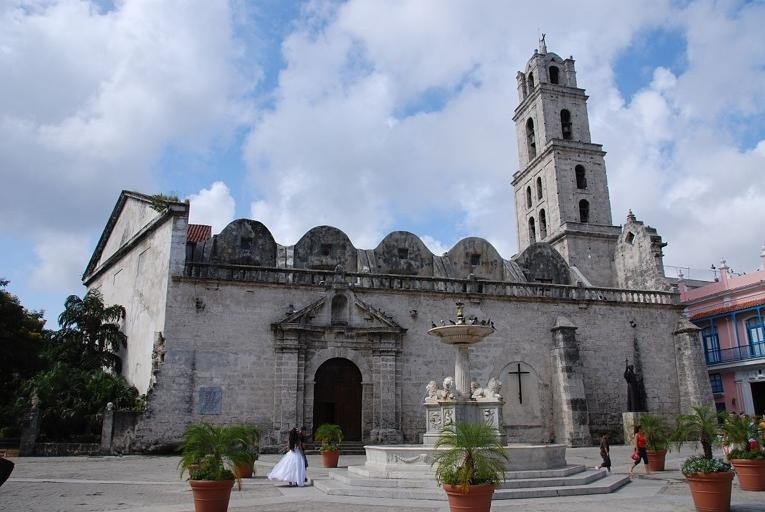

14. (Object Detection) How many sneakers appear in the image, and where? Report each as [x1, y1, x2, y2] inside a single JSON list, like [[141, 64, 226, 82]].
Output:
[[594, 465, 600, 469]]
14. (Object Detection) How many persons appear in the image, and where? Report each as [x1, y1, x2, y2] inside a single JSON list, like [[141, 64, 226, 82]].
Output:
[[267, 427, 306, 486], [623, 364, 644, 411], [721, 410, 765, 470], [595, 431, 612, 471], [629, 425, 650, 474], [298, 425, 308, 482]]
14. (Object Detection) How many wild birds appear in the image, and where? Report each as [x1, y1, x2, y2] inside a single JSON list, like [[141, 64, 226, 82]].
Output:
[[432, 317, 496, 331]]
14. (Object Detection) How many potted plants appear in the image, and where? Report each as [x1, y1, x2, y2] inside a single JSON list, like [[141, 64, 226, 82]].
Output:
[[315, 420, 345, 467], [427, 413, 512, 512], [175, 418, 261, 512], [637, 397, 764, 512]]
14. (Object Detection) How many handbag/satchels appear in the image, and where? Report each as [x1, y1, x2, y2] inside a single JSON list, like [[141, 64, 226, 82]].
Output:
[[631, 452, 641, 461]]
